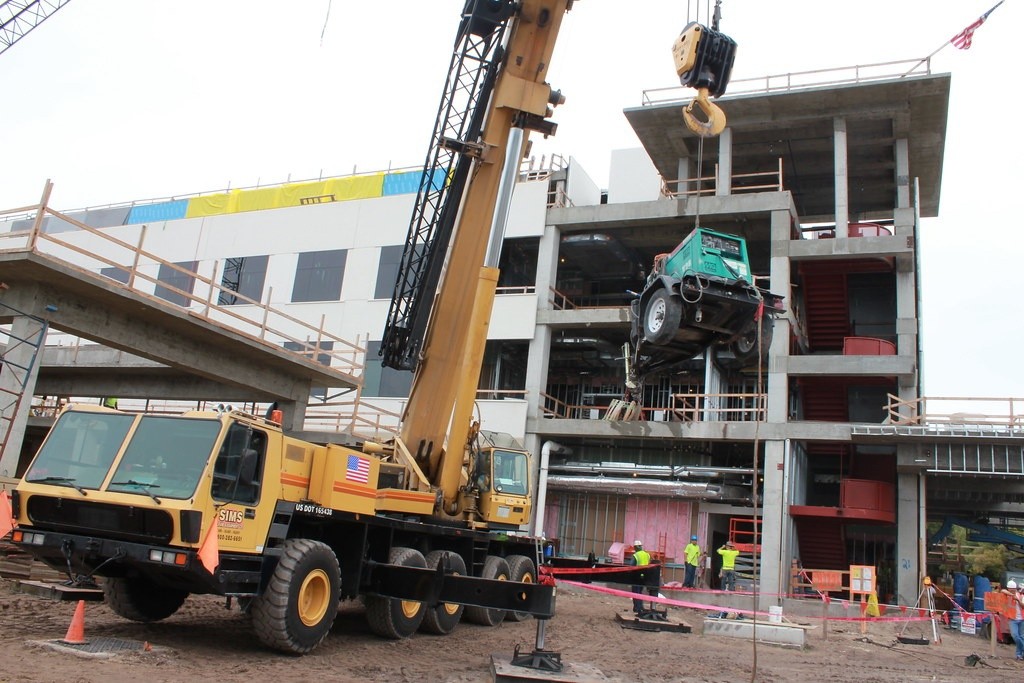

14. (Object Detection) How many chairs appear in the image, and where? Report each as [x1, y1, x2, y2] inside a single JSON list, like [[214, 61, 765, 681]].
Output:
[[216, 455, 256, 504]]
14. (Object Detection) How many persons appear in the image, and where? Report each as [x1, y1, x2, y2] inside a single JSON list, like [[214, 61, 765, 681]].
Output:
[[717, 542, 740, 591], [630, 541, 650, 612], [1001, 580, 1024, 659], [682, 535, 707, 589]]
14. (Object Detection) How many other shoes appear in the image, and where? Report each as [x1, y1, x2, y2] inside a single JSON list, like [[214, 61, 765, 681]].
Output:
[[1017, 655, 1023, 660]]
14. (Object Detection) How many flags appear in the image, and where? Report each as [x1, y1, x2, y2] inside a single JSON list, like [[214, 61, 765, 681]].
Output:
[[950, 1, 1006, 50]]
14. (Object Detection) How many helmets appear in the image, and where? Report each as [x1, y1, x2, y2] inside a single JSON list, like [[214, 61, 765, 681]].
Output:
[[633, 541, 642, 546], [1007, 580, 1017, 589], [691, 535, 697, 540], [725, 542, 734, 547]]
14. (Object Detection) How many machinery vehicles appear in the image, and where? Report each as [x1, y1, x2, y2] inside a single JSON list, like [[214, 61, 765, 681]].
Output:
[[613, 225, 791, 404], [6, 0, 737, 675]]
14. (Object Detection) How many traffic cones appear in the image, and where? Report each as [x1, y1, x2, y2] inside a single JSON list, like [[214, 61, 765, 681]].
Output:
[[57, 599, 91, 646]]
